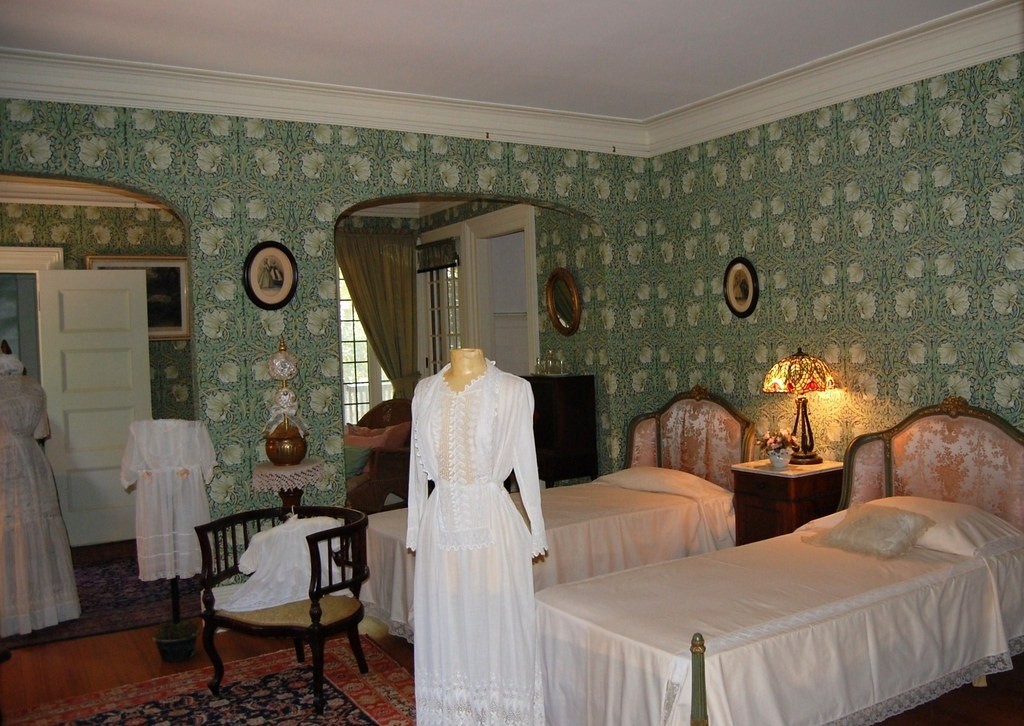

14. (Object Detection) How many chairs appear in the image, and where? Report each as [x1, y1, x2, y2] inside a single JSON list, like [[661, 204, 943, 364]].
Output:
[[348, 397, 412, 512], [195, 503, 372, 716]]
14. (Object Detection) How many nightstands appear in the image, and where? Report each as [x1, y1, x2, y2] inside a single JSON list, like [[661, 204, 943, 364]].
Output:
[[730, 456, 842, 549]]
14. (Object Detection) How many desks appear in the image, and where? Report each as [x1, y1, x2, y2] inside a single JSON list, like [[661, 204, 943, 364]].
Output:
[[254, 457, 325, 523]]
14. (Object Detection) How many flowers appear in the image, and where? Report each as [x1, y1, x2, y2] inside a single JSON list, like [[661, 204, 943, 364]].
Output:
[[754, 425, 797, 458]]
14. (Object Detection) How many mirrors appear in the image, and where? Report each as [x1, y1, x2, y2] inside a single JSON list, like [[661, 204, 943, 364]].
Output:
[[544, 266, 583, 337]]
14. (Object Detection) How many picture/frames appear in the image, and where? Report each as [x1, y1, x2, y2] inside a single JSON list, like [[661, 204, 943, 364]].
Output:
[[242, 240, 299, 311], [87, 254, 192, 342], [722, 255, 760, 320]]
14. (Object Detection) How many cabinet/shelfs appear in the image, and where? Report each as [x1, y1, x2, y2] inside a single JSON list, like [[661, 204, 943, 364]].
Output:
[[519, 373, 601, 487]]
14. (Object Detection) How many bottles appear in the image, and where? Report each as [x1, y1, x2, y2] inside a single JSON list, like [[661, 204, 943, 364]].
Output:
[[536, 350, 569, 374], [263, 414, 310, 466]]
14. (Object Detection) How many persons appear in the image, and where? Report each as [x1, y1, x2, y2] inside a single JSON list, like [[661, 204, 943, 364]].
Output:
[[406, 348, 548, 726], [0, 354, 81, 663], [269, 260, 283, 286], [258, 257, 274, 288]]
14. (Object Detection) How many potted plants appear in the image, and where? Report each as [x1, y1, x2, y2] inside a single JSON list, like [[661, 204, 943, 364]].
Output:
[[149, 619, 199, 664]]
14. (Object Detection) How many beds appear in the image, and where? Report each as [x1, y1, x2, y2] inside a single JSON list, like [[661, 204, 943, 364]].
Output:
[[535, 396, 1024, 726], [364, 382, 758, 649]]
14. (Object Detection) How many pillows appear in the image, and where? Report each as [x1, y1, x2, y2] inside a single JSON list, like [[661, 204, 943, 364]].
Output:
[[593, 465, 727, 502], [345, 433, 388, 473], [346, 422, 411, 448], [802, 500, 936, 560], [344, 443, 374, 479], [795, 494, 1024, 558]]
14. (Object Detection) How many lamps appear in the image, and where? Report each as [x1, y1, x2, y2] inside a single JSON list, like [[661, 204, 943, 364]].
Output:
[[263, 336, 309, 467], [762, 346, 837, 465]]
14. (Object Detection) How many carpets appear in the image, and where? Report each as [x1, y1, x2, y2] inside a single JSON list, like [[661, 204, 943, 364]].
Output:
[[0, 632, 417, 726]]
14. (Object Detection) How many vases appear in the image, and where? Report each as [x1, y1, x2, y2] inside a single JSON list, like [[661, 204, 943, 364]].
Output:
[[769, 447, 793, 468]]
